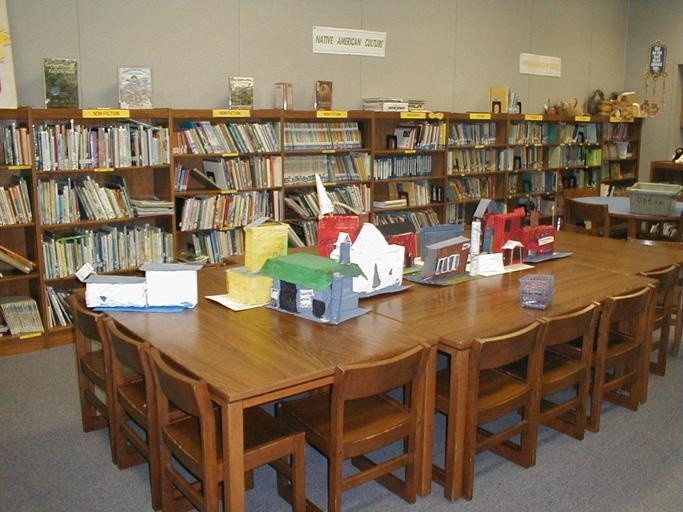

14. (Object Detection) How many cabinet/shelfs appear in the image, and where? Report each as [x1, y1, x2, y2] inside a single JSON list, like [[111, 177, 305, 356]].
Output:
[[446, 113, 507, 229], [282, 110, 373, 248], [170, 107, 283, 269], [560, 117, 602, 190], [0, 110, 175, 356], [600, 118, 641, 191], [371, 111, 447, 230], [649, 159, 683, 184], [505, 115, 561, 226]]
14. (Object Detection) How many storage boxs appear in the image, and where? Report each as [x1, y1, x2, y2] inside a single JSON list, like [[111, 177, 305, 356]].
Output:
[[625, 181, 683, 217]]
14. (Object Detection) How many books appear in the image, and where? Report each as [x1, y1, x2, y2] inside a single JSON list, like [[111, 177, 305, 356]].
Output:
[[548, 146, 602, 168], [374, 208, 440, 230], [0, 295, 44, 338], [76, 209, 573, 325], [392, 120, 446, 150], [561, 122, 602, 143], [610, 163, 623, 180], [172, 121, 281, 155], [509, 121, 558, 145], [561, 167, 598, 189], [446, 203, 466, 226], [284, 184, 371, 218], [117, 65, 152, 110], [284, 152, 371, 186], [176, 228, 244, 265], [447, 175, 495, 202], [507, 147, 543, 171], [607, 124, 633, 140], [43, 58, 79, 108], [33, 119, 170, 171], [448, 122, 496, 145], [276, 82, 292, 109], [228, 76, 255, 110], [373, 181, 444, 209], [0, 122, 31, 166], [447, 149, 506, 173], [37, 175, 174, 225], [174, 190, 279, 233], [362, 98, 428, 113], [42, 221, 173, 282], [0, 245, 36, 278], [0, 175, 32, 225], [614, 141, 634, 158], [373, 154, 432, 180], [489, 87, 521, 114], [508, 171, 558, 195], [283, 122, 362, 152], [315, 81, 333, 111], [175, 154, 283, 192], [517, 195, 556, 216], [46, 285, 72, 329], [286, 220, 318, 248]]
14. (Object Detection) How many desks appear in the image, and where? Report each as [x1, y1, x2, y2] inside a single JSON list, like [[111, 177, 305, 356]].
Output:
[[73, 264, 439, 512], [555, 231, 682, 391], [360, 257, 659, 503], [573, 196, 683, 240]]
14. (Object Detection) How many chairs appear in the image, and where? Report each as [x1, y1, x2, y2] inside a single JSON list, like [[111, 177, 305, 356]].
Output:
[[145, 347, 305, 512], [560, 199, 612, 235], [403, 318, 545, 500], [68, 294, 142, 466], [670, 261, 683, 358], [476, 301, 601, 468], [275, 340, 431, 512], [102, 316, 254, 512], [638, 261, 679, 377], [542, 284, 655, 433]]
